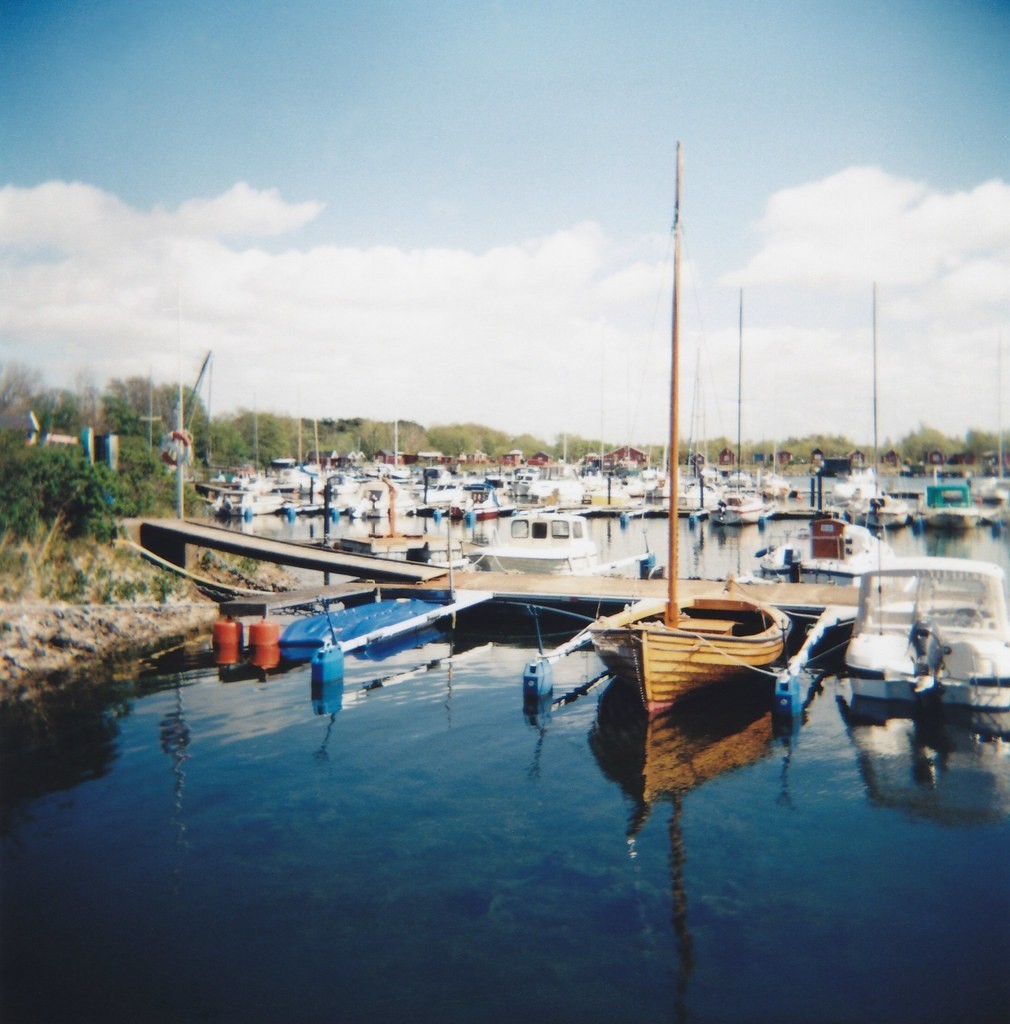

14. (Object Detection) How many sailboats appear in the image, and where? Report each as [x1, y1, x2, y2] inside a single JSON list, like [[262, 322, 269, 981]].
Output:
[[585, 136, 794, 715], [587, 666, 808, 1023]]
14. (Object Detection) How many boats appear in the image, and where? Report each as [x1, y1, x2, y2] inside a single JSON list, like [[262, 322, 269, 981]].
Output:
[[460, 510, 649, 578], [754, 517, 895, 587], [844, 558, 1010, 711], [211, 283, 1009, 533]]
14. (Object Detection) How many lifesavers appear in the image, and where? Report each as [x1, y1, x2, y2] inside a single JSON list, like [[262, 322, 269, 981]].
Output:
[[159, 431, 191, 465]]
